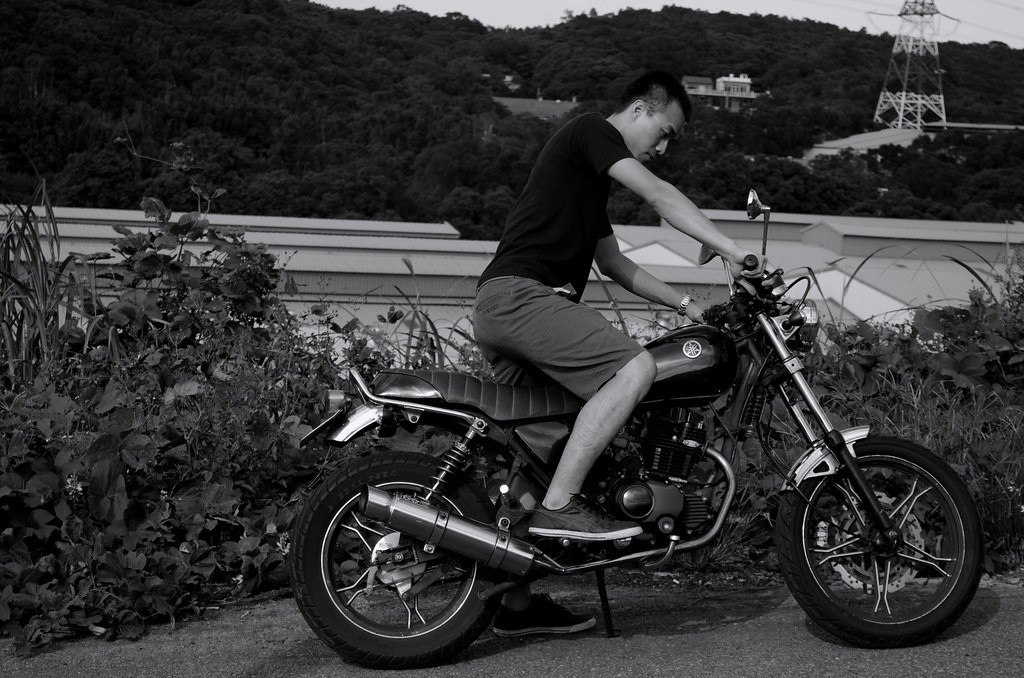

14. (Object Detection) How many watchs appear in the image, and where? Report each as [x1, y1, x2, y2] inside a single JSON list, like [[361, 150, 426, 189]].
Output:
[[677, 294, 695, 317]]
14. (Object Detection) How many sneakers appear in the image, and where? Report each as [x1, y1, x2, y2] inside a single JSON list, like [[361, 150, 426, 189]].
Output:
[[529, 493, 644, 541], [492, 593, 597, 638]]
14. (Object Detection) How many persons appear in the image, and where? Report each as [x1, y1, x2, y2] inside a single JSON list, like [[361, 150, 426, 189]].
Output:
[[473, 70, 768, 638]]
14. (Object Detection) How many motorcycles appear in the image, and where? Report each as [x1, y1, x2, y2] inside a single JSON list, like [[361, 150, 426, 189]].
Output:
[[288, 188, 985, 670]]
